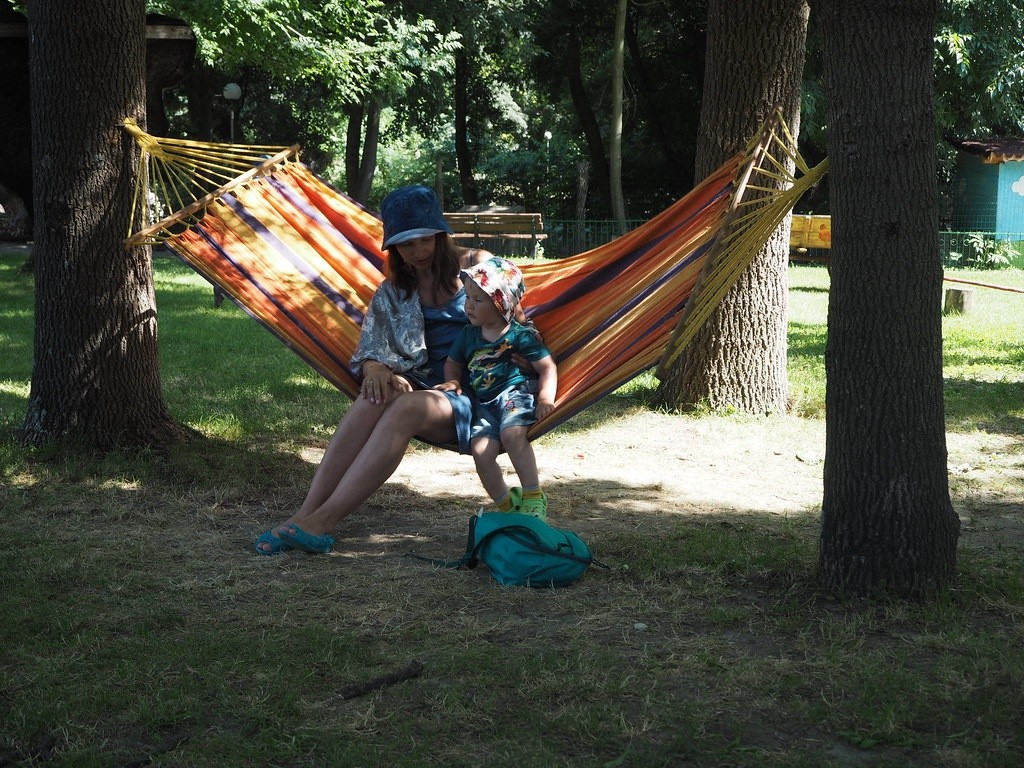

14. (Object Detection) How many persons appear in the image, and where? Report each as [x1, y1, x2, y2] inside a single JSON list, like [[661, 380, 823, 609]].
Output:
[[256, 186, 557, 555]]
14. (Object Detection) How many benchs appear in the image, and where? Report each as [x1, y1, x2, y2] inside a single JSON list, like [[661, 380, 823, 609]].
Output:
[[442, 212, 548, 259], [789, 214, 832, 263]]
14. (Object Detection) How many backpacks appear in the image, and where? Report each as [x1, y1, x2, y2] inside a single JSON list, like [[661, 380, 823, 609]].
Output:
[[456, 512, 593, 587]]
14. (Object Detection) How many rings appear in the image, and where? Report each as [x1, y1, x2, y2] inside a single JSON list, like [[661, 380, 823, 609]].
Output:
[[366, 380, 372, 384]]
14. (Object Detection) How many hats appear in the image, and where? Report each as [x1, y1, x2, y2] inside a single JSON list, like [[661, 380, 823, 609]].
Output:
[[381, 185, 454, 251], [460, 256, 525, 325]]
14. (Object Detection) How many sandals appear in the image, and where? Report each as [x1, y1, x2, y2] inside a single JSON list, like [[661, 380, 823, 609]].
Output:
[[517, 493, 547, 521], [279, 523, 335, 553], [255, 529, 285, 554], [498, 487, 522, 512]]
